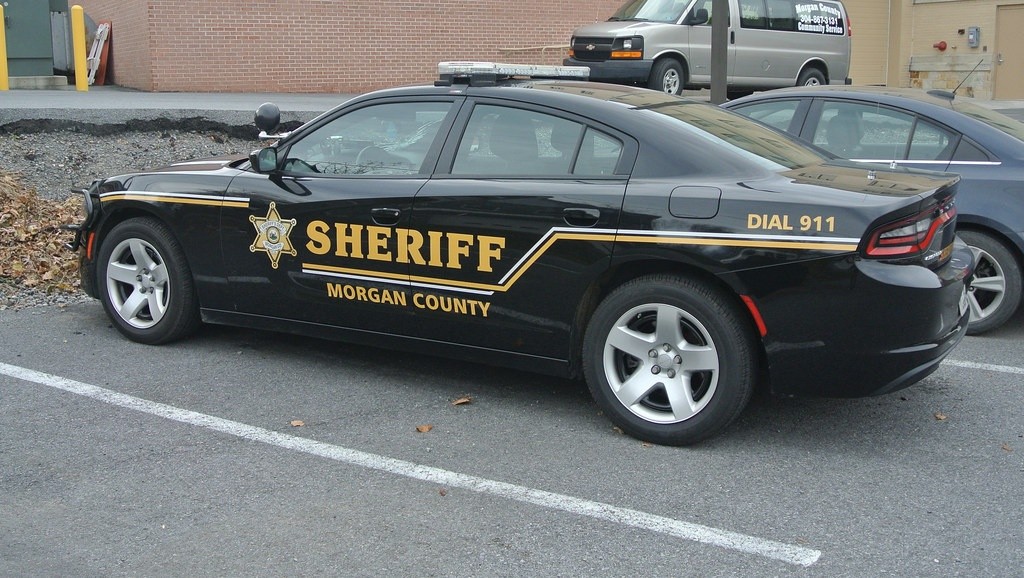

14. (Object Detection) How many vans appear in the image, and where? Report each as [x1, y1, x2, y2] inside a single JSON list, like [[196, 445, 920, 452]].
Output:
[[561, 0, 854, 116]]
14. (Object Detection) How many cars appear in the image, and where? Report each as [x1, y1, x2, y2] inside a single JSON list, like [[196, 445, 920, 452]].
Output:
[[710, 85, 1023, 337], [73, 58, 978, 447]]
